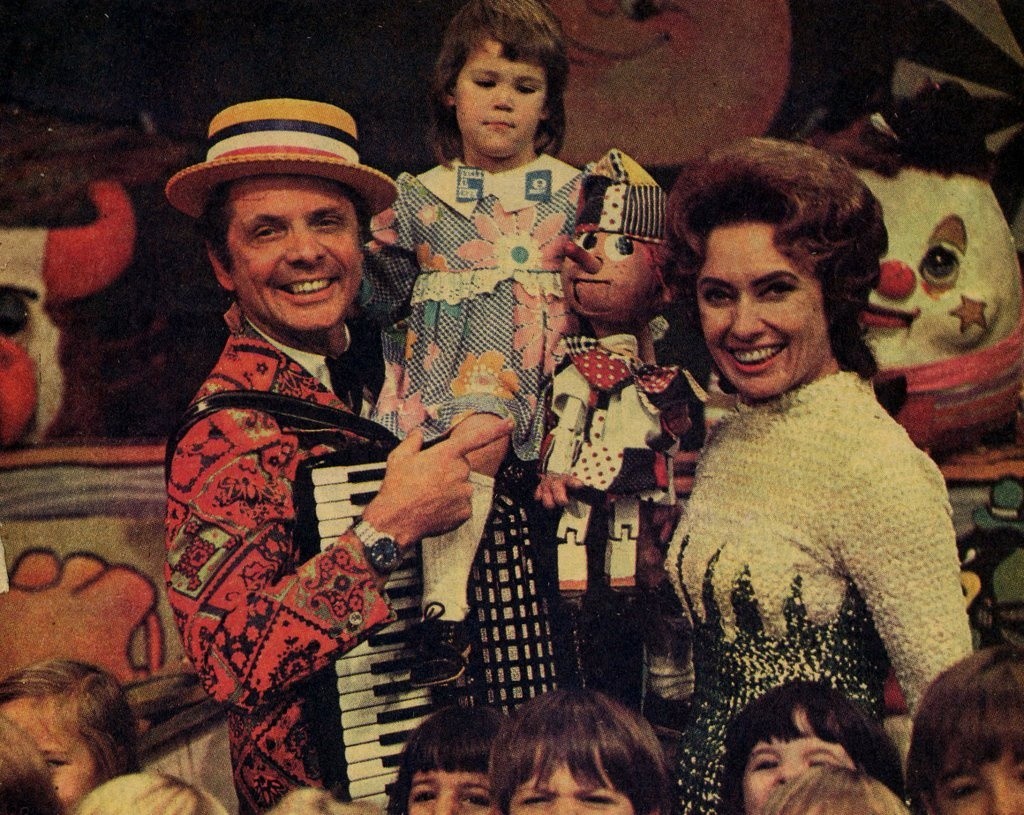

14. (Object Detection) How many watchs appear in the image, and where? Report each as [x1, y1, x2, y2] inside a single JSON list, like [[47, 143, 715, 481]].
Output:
[[351, 517, 406, 573]]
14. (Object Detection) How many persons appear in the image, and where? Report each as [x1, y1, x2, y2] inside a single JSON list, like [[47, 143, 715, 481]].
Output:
[[0, 82, 1024, 815], [225, 0, 691, 687], [531, 148, 686, 600]]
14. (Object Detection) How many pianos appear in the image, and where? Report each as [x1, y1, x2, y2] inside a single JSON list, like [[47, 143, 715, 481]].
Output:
[[292, 439, 584, 814]]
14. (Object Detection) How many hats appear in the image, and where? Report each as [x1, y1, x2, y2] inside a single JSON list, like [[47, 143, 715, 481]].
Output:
[[165, 97, 398, 219]]
